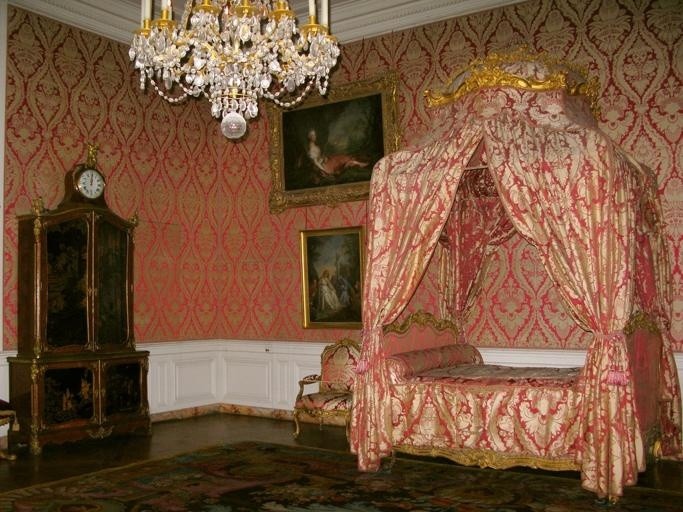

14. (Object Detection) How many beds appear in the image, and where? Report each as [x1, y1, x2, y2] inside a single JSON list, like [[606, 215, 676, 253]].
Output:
[[364, 310, 665, 507]]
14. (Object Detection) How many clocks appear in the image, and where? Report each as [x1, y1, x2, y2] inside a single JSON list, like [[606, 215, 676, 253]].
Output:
[[63, 142, 107, 207]]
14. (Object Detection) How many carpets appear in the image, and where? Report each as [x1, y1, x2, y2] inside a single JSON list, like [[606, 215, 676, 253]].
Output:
[[0, 438, 683, 512]]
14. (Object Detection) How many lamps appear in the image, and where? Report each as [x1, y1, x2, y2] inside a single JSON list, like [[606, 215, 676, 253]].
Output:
[[129, 0, 340, 141]]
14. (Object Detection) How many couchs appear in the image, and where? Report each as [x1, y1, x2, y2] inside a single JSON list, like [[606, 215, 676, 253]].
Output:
[[292, 336, 361, 442]]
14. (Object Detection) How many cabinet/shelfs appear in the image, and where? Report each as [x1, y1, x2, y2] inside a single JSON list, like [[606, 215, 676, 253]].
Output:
[[9, 194, 154, 456]]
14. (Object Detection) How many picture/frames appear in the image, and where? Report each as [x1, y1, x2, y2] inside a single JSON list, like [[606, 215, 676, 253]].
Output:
[[265, 71, 402, 215], [299, 226, 364, 330]]
